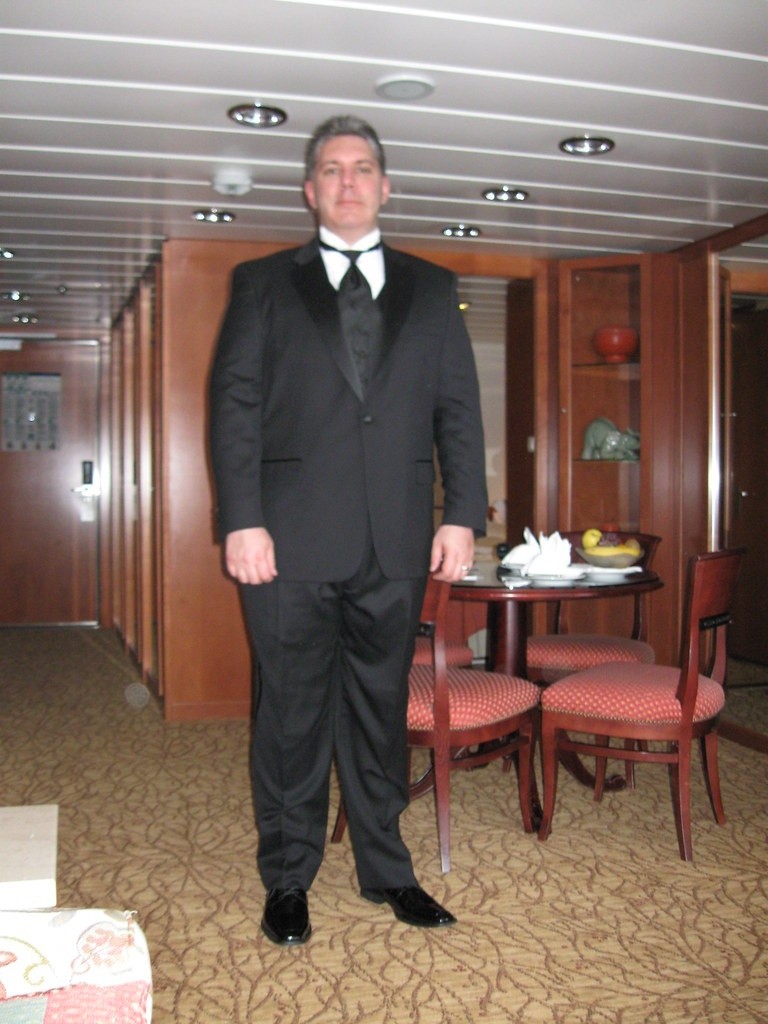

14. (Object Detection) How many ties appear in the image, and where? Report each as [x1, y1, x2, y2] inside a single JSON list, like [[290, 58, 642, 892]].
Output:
[[320, 239, 383, 395]]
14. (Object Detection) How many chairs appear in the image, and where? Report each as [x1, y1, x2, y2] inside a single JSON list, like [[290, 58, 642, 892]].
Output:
[[331, 530, 750, 865], [0, 907, 155, 1024]]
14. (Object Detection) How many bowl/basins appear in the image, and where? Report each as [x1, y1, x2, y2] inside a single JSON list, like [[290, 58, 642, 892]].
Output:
[[575, 547, 644, 566]]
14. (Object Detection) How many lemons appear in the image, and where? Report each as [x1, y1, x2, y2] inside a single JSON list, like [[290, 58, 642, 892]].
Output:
[[585, 529, 601, 546]]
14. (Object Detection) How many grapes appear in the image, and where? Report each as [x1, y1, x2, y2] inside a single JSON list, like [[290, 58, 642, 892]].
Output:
[[598, 531, 619, 548]]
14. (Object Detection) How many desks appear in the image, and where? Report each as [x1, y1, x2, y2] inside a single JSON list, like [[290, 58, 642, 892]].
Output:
[[441, 557, 664, 837]]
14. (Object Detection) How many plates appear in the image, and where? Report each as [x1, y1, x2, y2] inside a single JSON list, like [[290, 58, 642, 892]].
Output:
[[572, 562, 643, 581], [528, 573, 586, 588]]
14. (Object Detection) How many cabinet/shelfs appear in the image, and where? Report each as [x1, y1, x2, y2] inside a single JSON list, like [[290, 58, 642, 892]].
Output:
[[556, 253, 653, 653]]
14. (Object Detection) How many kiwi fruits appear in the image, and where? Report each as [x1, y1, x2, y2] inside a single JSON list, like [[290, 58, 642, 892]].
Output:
[[626, 539, 639, 547]]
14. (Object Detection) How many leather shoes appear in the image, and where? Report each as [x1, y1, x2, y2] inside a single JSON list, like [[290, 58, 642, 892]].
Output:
[[360, 884, 458, 928], [261, 887, 311, 945]]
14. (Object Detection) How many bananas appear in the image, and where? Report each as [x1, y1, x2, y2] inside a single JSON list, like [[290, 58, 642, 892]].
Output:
[[585, 545, 638, 557]]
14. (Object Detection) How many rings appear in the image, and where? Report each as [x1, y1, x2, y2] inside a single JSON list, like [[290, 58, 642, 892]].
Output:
[[461, 565, 469, 571]]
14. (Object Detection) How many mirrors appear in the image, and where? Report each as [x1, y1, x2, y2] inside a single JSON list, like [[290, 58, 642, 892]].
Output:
[[707, 232, 768, 742]]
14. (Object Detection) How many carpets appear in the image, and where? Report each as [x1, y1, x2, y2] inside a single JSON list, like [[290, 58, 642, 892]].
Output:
[[0, 803, 59, 909]]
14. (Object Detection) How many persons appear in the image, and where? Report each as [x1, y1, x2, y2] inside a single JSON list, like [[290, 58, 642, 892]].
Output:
[[206, 114, 490, 945]]
